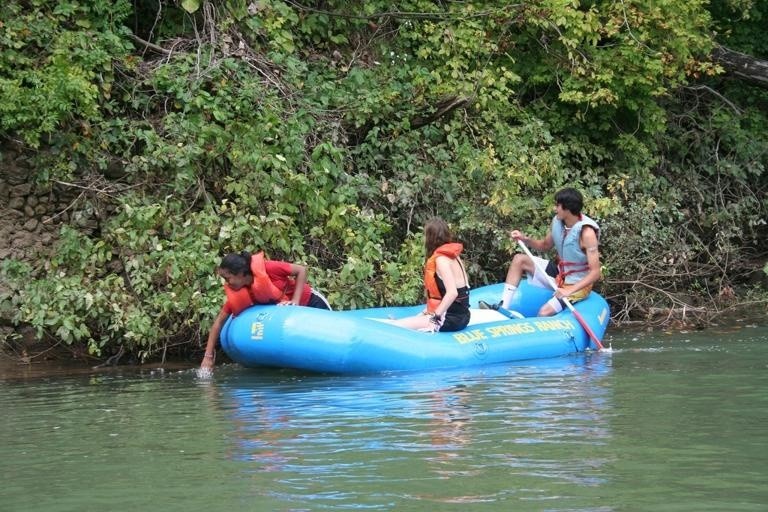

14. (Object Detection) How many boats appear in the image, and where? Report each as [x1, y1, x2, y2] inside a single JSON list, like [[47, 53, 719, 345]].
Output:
[[220, 276, 611, 373]]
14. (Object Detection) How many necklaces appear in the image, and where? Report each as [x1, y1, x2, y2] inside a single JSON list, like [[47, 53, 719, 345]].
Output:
[[562, 224, 573, 231]]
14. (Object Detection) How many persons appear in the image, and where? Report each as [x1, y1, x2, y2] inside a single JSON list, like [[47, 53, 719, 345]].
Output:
[[199, 250, 333, 378], [478, 188, 602, 317], [396, 215, 471, 331]]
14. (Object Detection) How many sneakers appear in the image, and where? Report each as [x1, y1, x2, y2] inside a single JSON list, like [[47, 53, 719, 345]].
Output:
[[478, 300, 503, 311]]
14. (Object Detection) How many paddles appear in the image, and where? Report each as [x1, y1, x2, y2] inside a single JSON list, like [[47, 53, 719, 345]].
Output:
[[512, 232, 603, 349]]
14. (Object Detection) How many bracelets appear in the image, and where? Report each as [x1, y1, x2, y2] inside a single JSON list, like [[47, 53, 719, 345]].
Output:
[[430, 311, 442, 322], [204, 351, 214, 359]]
